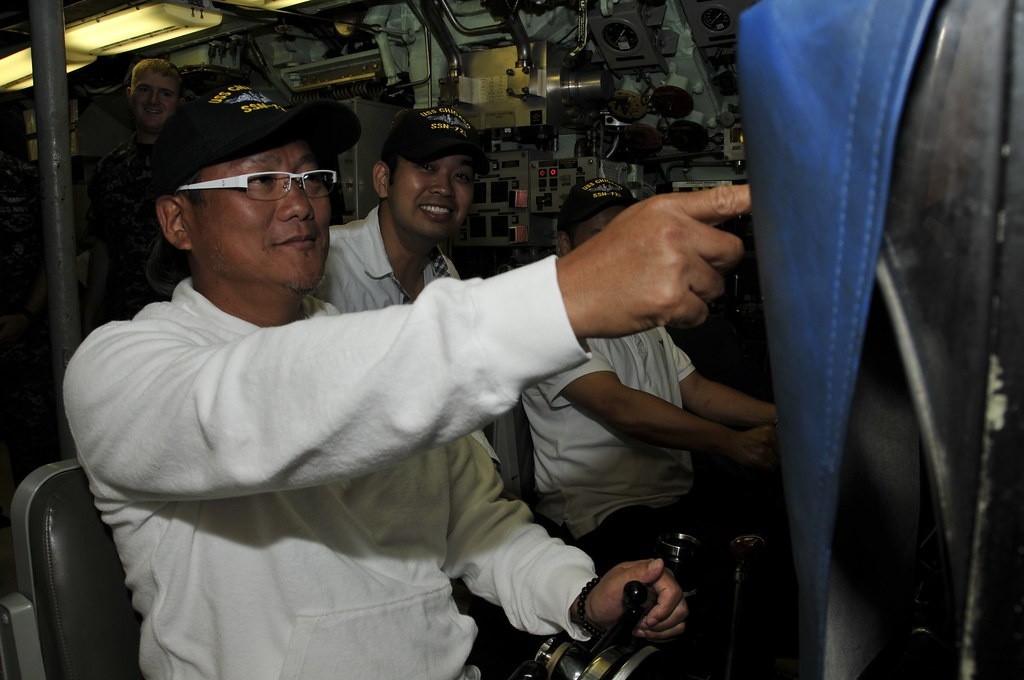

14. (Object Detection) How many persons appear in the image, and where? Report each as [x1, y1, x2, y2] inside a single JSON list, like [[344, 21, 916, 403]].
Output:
[[524, 179, 778, 573], [316, 106, 488, 314], [63, 81, 752, 680], [81, 59, 192, 338]]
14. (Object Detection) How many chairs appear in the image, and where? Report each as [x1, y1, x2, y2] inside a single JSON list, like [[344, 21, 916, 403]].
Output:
[[0, 458, 140, 680]]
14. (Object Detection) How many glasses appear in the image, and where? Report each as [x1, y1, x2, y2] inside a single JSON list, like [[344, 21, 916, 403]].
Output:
[[173, 169, 339, 201]]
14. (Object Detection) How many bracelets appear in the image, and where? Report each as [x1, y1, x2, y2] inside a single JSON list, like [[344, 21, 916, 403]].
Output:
[[577, 575, 607, 639]]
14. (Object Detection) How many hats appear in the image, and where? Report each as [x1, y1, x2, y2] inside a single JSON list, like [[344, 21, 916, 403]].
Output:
[[386, 106, 491, 175], [149, 83, 362, 202], [556, 177, 641, 231]]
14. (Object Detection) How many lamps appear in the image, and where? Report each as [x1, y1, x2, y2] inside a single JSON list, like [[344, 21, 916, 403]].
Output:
[[0, 46, 97, 92], [215, 0, 310, 11], [64, 0, 223, 56]]
